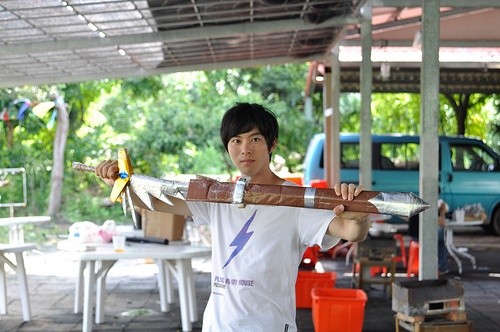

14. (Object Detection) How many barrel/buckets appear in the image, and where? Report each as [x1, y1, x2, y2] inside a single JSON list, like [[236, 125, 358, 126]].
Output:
[[311, 288, 367, 332], [296, 271, 336, 308]]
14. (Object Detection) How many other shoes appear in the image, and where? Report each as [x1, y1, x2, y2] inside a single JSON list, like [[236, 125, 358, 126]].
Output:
[[439, 269, 449, 276]]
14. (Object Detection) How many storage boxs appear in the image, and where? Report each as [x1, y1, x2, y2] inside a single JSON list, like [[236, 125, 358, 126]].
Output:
[[142, 208, 185, 241], [310, 287, 367, 332], [292, 271, 337, 308]]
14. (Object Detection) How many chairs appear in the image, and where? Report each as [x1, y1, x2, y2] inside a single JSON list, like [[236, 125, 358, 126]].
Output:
[[351, 233, 397, 299]]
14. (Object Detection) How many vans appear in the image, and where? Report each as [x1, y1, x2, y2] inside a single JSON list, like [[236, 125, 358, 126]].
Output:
[[302, 132, 500, 236]]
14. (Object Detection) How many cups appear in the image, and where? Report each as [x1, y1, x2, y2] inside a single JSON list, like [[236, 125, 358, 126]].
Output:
[[455, 210, 465, 222], [113, 236, 126, 252]]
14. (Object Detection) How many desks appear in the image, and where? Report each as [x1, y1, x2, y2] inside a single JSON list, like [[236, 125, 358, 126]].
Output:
[[58, 240, 191, 314], [70, 245, 211, 332], [0, 243, 37, 322], [0, 216, 51, 260], [370, 214, 483, 274]]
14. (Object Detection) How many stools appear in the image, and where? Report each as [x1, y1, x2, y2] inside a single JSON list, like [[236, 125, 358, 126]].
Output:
[[406, 240, 419, 278]]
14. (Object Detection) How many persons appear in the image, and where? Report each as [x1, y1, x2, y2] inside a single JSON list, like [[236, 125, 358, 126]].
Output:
[[408, 186, 449, 276], [96, 102, 372, 332]]
